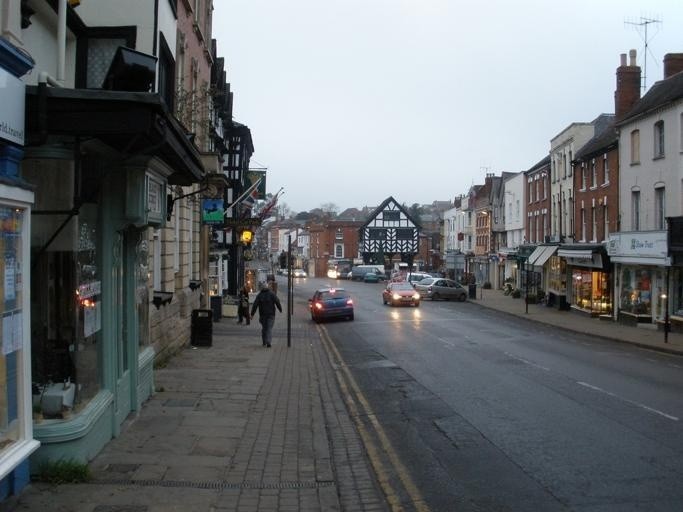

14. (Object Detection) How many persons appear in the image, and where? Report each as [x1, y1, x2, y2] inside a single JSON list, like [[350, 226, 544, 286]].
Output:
[[237, 276, 282, 348]]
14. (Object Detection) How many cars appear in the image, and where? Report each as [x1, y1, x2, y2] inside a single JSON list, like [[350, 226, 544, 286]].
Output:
[[309, 288, 353, 324], [277, 269, 305, 278], [347, 262, 467, 306]]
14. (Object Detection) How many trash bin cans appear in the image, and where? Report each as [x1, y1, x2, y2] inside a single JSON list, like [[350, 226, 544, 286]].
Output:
[[211, 296, 222, 322], [469, 284, 476, 299], [191, 309, 213, 347]]
